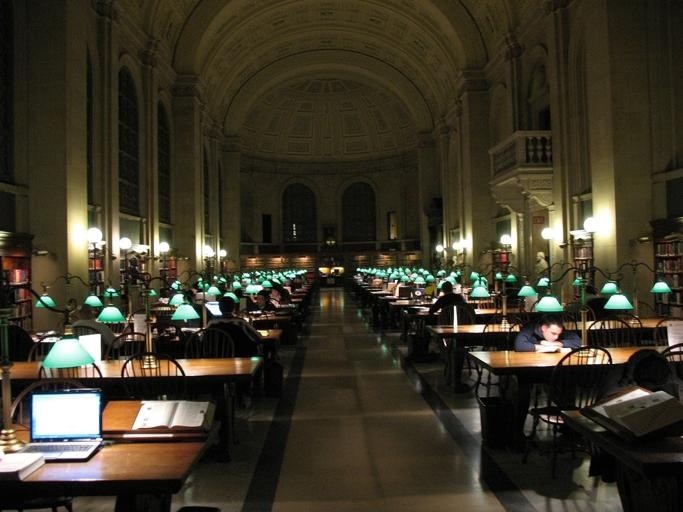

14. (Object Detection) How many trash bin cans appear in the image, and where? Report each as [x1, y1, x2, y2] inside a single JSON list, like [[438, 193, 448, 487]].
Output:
[[407, 332, 431, 362], [477, 395, 515, 442], [264, 360, 284, 398], [177, 506, 221, 512]]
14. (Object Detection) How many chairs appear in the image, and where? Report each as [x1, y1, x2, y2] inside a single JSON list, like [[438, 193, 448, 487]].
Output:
[[348, 266, 682, 512], [0, 268, 319, 512]]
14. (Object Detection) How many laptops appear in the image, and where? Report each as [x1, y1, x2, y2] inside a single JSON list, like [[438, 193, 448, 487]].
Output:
[[16, 387, 104, 463], [207, 301, 223, 316]]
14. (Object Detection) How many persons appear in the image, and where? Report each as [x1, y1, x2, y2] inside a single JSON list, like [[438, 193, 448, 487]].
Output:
[[429, 284, 477, 325], [177, 273, 307, 357], [73, 304, 128, 357], [533, 252, 547, 274], [453, 256, 458, 266], [514, 317, 582, 353]]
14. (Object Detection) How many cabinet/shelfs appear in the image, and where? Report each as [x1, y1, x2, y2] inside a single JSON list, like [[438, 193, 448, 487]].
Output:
[[647, 215, 681, 321], [567, 227, 595, 297], [86, 247, 177, 288], [0, 229, 35, 335], [488, 239, 513, 279]]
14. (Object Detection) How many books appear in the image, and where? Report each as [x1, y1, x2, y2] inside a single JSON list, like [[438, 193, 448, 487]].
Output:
[[130, 400, 215, 432], [536, 347, 571, 353], [593, 388, 680, 436], [7, 270, 34, 332], [1, 452, 47, 480], [666, 321, 683, 352], [654, 242, 683, 316]]
